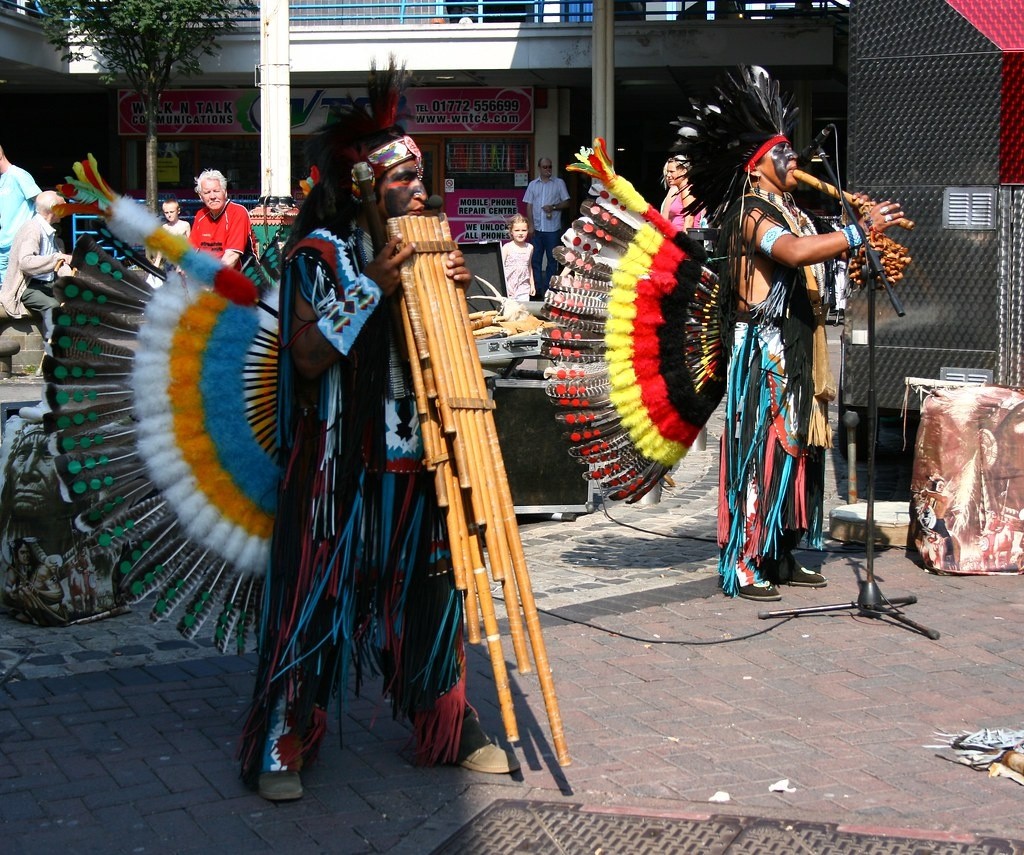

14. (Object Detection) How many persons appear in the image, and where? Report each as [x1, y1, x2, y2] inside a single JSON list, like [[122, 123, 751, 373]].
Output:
[[0, 190, 78, 337], [660, 153, 709, 236], [175, 168, 259, 295], [715, 135, 905, 600], [0, 143, 42, 292], [502, 212, 536, 300], [523, 157, 571, 299], [242, 135, 522, 800], [145, 198, 190, 288]]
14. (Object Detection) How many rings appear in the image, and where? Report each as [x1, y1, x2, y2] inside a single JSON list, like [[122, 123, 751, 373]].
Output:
[[883, 215, 892, 222], [880, 206, 889, 214]]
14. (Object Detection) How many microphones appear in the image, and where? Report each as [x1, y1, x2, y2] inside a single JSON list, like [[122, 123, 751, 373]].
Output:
[[429, 195, 443, 213], [797, 124, 833, 169]]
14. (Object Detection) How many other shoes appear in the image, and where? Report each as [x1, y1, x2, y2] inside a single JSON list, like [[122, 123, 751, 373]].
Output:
[[738, 579, 781, 600], [259, 770, 302, 802], [789, 564, 828, 587], [461, 742, 521, 774]]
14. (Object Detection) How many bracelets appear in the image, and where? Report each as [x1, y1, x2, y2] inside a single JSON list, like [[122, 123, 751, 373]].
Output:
[[840, 224, 863, 250], [551, 204, 557, 210]]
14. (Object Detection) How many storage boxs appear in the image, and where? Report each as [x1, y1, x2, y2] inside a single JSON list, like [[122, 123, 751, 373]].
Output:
[[485, 375, 595, 515]]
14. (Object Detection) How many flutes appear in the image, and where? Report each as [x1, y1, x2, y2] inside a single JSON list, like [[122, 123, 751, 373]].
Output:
[[387, 212, 572, 770], [793, 169, 915, 232]]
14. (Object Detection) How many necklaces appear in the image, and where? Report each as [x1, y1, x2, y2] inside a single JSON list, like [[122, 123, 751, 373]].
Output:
[[749, 185, 826, 298]]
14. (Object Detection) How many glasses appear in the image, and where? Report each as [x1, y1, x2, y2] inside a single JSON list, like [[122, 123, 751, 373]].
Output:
[[541, 166, 552, 169]]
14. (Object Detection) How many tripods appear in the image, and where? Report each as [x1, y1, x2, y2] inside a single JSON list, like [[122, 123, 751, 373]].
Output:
[[758, 148, 941, 640]]
